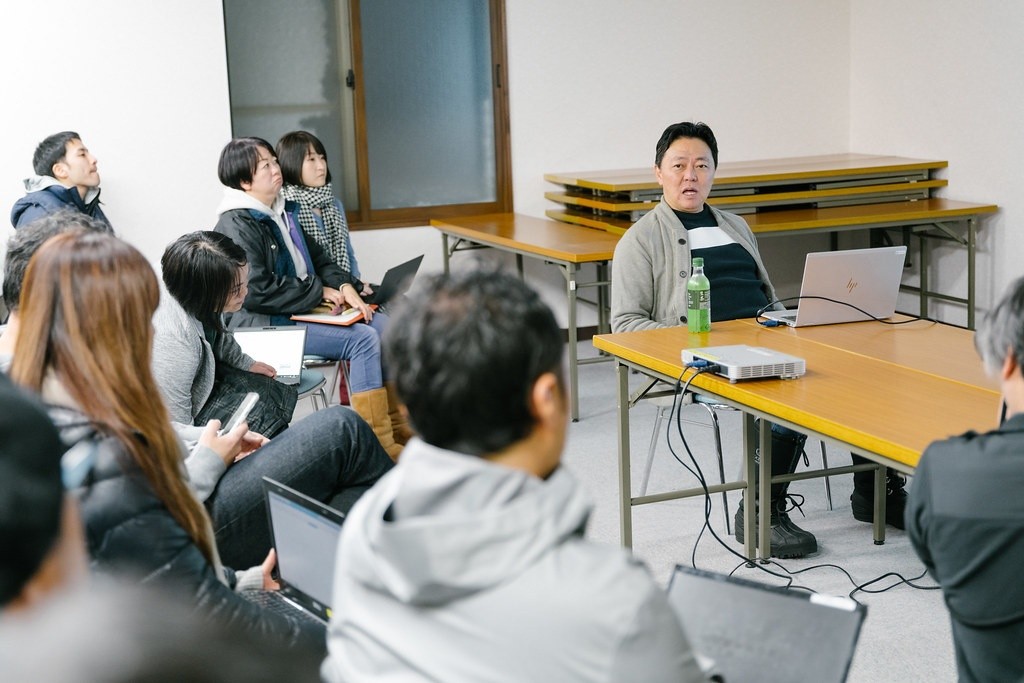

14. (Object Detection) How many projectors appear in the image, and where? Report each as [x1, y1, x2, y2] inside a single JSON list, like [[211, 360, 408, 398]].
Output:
[[681, 345, 806, 383]]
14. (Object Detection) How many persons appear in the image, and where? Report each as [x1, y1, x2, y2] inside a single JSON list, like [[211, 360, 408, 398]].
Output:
[[0, 226, 330, 683], [0, 131, 416, 570], [611, 122, 911, 560], [321, 267, 705, 681], [0, 369, 326, 683], [905, 276, 1024, 683]]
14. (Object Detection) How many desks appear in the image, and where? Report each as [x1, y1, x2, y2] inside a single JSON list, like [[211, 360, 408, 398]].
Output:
[[426, 152, 1023, 570]]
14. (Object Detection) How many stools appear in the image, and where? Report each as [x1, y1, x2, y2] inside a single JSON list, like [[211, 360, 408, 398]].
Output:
[[291, 353, 352, 413], [635, 390, 742, 536]]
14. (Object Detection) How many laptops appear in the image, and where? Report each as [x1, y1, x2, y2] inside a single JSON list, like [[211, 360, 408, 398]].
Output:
[[663, 564, 869, 683], [761, 246, 908, 328], [361, 254, 425, 304], [231, 325, 308, 386], [228, 476, 346, 634]]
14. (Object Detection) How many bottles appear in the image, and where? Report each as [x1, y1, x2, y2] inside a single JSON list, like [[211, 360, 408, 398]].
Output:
[[687, 256, 711, 334]]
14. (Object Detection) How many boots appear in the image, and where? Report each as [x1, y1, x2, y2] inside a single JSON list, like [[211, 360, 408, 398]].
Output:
[[850, 453, 911, 529], [350, 386, 404, 461], [386, 388, 415, 444], [735, 420, 818, 557]]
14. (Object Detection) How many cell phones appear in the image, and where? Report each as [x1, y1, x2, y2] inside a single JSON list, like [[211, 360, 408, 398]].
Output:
[[220, 391, 259, 438]]
